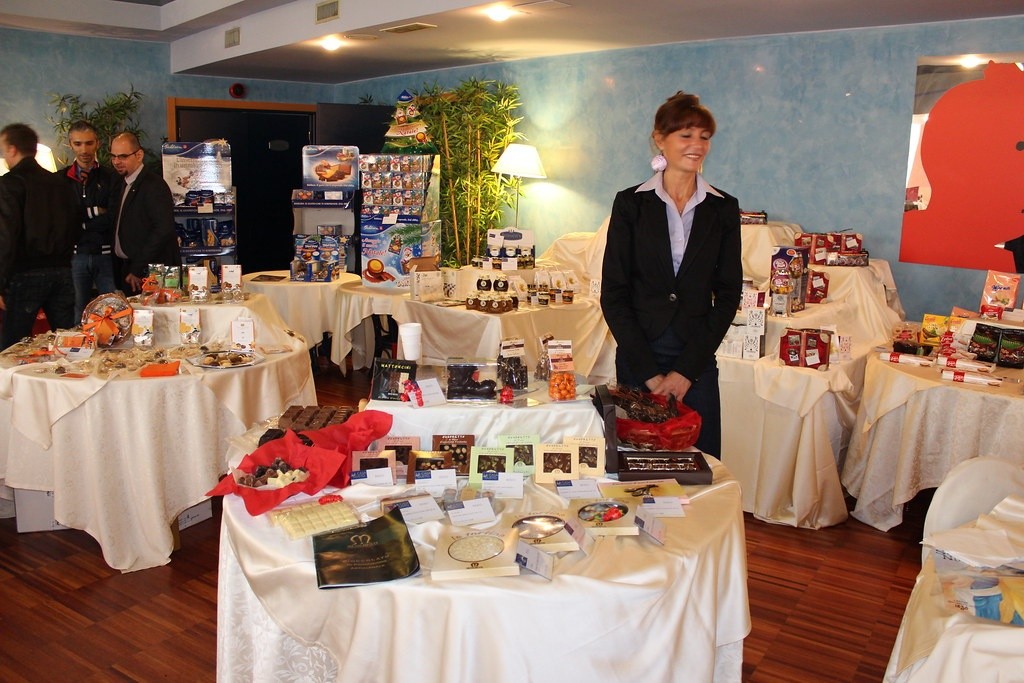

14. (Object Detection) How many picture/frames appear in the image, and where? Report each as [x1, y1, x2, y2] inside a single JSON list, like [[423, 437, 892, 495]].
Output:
[[353, 434, 605, 484]]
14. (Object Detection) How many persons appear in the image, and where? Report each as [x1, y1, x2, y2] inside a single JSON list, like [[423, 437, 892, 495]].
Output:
[[51, 119, 122, 325], [599, 93, 742, 460], [0, 123, 79, 350], [110, 132, 184, 298]]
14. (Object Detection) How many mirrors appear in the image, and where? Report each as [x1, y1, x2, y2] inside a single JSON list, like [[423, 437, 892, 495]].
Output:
[[898, 53, 1024, 273]]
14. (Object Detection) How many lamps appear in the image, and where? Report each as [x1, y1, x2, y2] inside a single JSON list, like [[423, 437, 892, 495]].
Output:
[[491, 145, 547, 228]]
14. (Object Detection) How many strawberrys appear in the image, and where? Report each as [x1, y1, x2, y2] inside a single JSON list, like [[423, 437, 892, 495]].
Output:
[[601, 507, 624, 521]]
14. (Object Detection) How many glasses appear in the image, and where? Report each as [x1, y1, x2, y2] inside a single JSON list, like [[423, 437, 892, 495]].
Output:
[[108, 148, 139, 161]]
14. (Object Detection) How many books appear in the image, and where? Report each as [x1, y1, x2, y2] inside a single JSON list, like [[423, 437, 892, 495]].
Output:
[[597, 479, 690, 506], [311, 508, 423, 590]]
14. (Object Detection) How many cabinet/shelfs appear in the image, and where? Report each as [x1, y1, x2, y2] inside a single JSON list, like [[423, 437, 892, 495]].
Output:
[[290, 146, 362, 279], [162, 143, 238, 293]]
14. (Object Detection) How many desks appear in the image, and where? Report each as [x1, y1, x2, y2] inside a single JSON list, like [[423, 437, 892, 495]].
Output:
[[216, 397, 753, 683], [1, 291, 318, 574], [882, 520, 1024, 683], [840, 318, 1024, 531], [535, 217, 905, 530], [239, 271, 610, 377]]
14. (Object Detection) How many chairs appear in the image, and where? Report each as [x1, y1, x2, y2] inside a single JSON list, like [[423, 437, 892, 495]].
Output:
[[921, 458, 1024, 567]]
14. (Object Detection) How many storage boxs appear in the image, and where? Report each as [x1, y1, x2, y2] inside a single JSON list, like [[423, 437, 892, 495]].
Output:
[[920, 307, 980, 346], [187, 218, 235, 286], [14, 489, 69, 532], [618, 451, 713, 484], [431, 499, 639, 580], [290, 156, 463, 301], [739, 246, 809, 360], [178, 500, 213, 531]]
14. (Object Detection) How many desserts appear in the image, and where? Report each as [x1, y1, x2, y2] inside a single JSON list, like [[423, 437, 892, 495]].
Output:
[[258, 405, 353, 449]]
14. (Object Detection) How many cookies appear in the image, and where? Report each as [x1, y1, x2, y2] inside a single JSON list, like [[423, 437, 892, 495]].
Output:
[[84, 342, 253, 376]]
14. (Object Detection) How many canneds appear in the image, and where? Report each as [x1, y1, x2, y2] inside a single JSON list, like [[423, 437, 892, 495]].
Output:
[[466, 274, 519, 315], [527, 284, 574, 304]]
